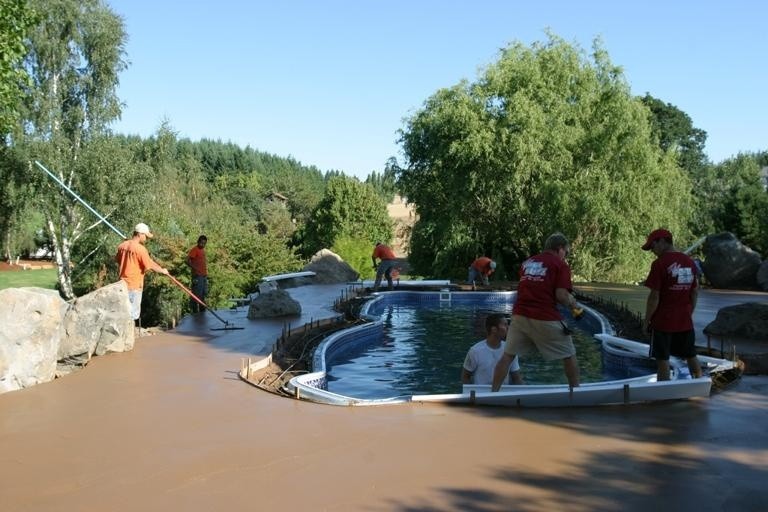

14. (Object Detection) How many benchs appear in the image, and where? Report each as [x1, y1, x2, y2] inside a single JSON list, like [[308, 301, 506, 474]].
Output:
[[347, 280, 451, 289]]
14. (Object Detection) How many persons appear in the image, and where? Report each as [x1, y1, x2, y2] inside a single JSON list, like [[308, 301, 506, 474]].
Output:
[[462, 313, 525, 385], [467, 256, 497, 285], [640, 228, 702, 381], [372, 243, 396, 290], [116, 222, 169, 322], [184, 235, 208, 313], [491, 232, 580, 392]]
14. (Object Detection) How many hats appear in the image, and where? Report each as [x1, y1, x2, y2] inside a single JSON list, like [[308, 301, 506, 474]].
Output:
[[489, 262, 497, 272], [641, 228, 672, 250], [134, 223, 153, 238]]
